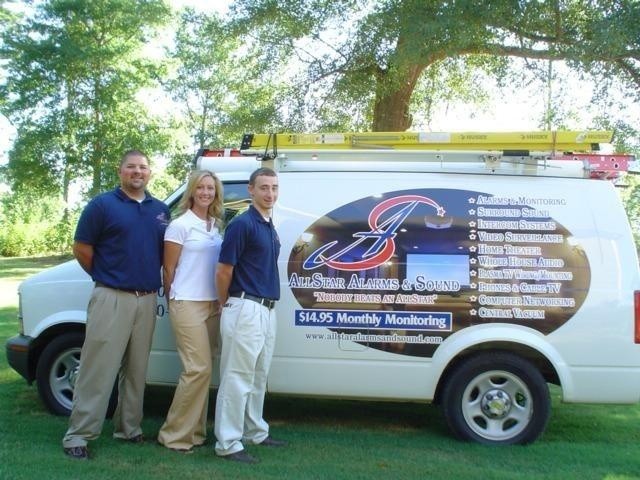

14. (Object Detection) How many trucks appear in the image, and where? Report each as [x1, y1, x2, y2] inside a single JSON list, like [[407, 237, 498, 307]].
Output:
[[4, 128, 638, 446]]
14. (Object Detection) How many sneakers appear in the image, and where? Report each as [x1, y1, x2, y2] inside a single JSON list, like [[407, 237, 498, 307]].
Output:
[[64, 446, 88, 461], [125, 434, 145, 444]]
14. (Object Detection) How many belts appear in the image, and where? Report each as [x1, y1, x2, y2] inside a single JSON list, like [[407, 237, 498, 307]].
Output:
[[95, 281, 157, 297], [228, 290, 275, 310]]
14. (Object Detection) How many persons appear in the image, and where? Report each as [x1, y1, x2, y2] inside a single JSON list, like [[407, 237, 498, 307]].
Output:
[[157, 169, 228, 451], [215, 166, 284, 464], [62, 149, 171, 459]]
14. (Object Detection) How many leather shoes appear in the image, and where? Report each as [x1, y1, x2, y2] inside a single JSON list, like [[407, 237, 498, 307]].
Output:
[[261, 435, 289, 446], [225, 451, 260, 464]]
